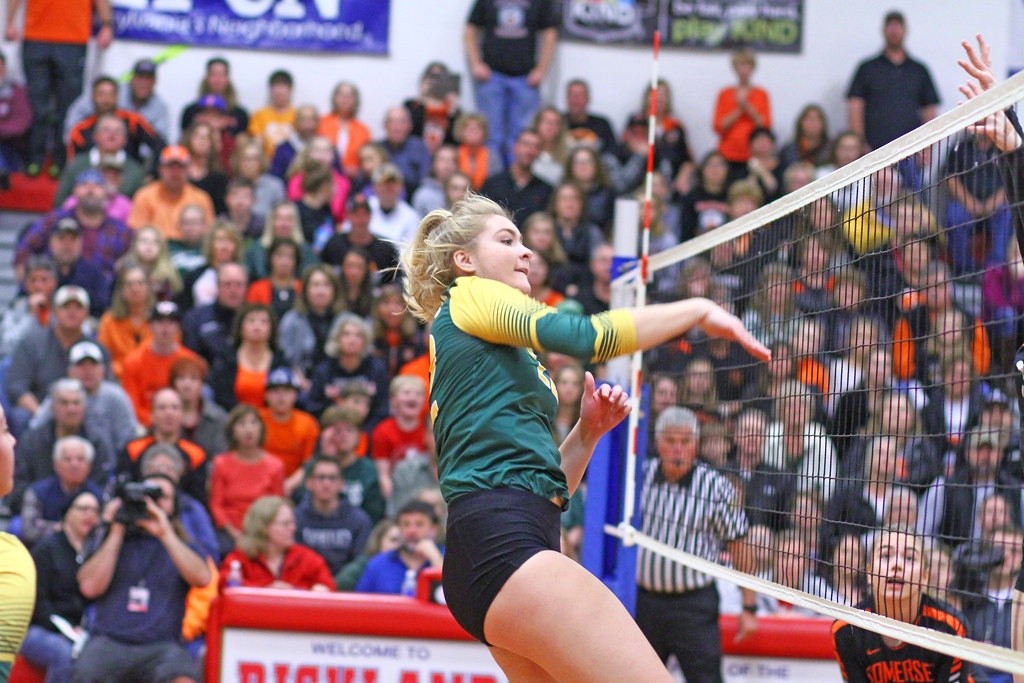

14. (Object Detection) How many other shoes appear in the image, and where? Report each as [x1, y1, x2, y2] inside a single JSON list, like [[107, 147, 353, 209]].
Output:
[[48, 163, 61, 179], [27, 157, 38, 174]]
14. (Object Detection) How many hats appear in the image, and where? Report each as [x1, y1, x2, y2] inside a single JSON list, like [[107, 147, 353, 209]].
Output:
[[625, 112, 649, 128], [53, 215, 82, 235], [345, 194, 373, 214], [266, 368, 303, 392], [146, 300, 181, 323], [371, 161, 403, 186], [69, 342, 102, 364], [55, 284, 91, 310], [134, 58, 157, 78], [159, 146, 190, 168], [967, 427, 1001, 452], [196, 93, 225, 113], [973, 384, 1009, 410]]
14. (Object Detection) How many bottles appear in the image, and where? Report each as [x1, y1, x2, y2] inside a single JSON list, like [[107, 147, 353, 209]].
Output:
[[228, 561, 241, 586], [401, 570, 416, 597]]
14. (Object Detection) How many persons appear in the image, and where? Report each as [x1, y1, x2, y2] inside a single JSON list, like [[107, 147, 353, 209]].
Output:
[[0, 0, 1024, 683], [379, 192, 773, 683]]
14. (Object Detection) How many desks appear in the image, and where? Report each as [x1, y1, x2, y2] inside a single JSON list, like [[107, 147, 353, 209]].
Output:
[[197, 584, 846, 683]]
